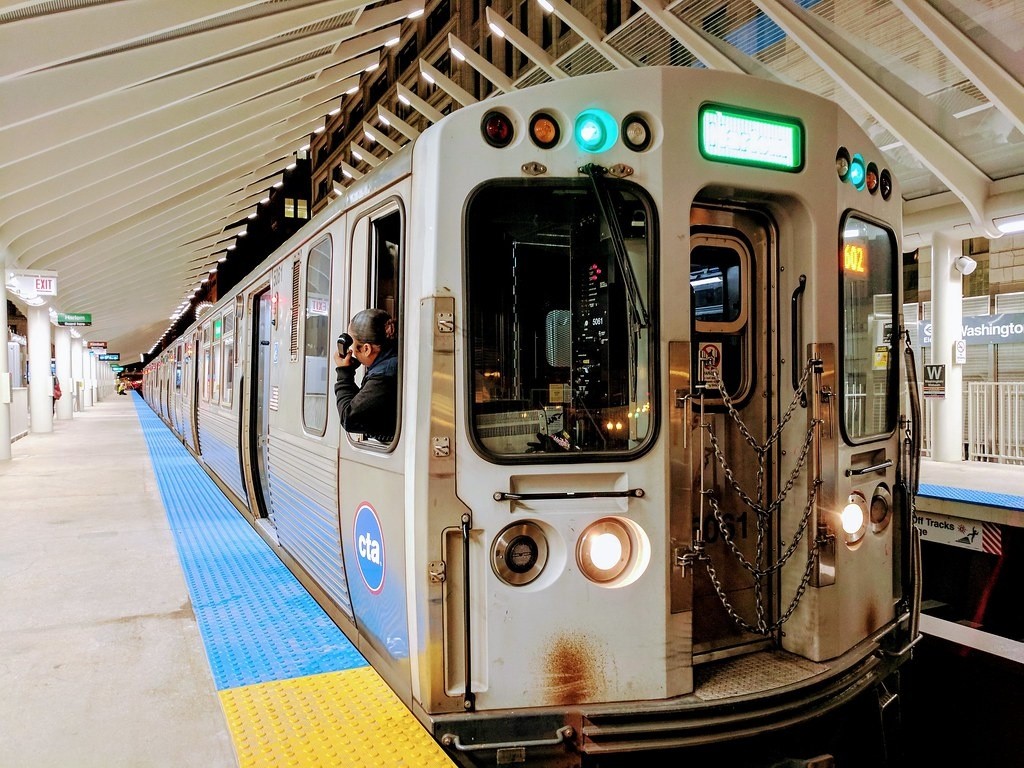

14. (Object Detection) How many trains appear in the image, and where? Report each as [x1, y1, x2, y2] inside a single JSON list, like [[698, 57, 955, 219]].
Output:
[[141, 64, 931, 763]]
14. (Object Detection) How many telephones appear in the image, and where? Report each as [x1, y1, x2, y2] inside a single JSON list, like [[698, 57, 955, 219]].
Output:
[[336, 333, 361, 370]]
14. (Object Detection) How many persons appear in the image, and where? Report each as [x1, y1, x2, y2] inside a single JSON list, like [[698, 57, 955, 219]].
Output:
[[334, 309, 398, 444], [52, 372, 59, 416], [117, 375, 131, 395]]
